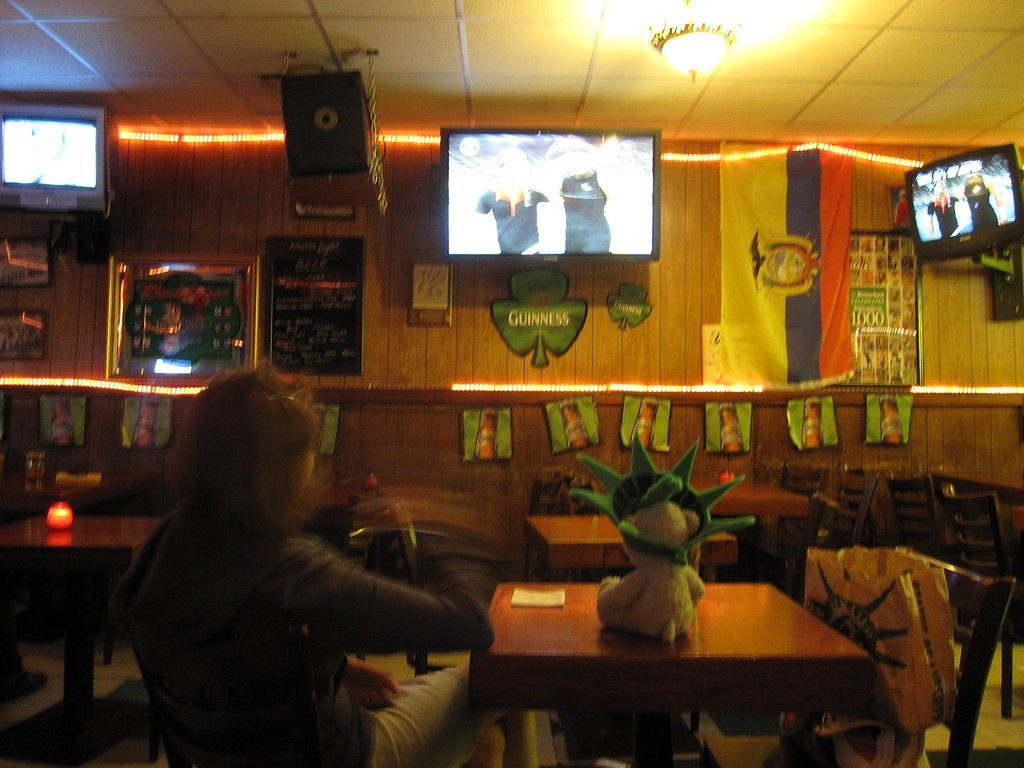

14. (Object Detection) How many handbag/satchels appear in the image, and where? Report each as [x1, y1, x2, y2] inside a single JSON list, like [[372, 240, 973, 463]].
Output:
[[800, 545, 957, 735]]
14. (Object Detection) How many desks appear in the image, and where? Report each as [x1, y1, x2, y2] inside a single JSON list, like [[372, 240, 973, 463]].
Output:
[[0, 515, 162, 768], [0, 468, 132, 515], [526, 513, 739, 579], [692, 479, 808, 582], [931, 472, 1024, 508], [467, 580, 876, 768]]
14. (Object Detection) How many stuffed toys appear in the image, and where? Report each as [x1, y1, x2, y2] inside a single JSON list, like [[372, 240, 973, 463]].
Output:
[[567, 432, 757, 646]]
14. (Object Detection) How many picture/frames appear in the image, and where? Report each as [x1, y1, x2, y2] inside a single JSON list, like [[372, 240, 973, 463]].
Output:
[[0, 235, 54, 289], [104, 252, 262, 387], [0, 308, 49, 361]]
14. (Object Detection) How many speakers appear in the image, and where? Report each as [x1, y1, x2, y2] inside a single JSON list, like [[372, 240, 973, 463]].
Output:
[[280, 71, 378, 203]]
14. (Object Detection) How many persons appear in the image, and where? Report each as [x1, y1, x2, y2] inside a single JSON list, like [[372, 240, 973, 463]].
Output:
[[475, 137, 613, 256], [926, 172, 1008, 241], [115, 360, 508, 768]]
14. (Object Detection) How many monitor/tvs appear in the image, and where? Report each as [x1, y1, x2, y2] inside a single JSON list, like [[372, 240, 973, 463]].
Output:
[[440, 127, 661, 261], [0, 104, 106, 213], [904, 143, 1024, 260]]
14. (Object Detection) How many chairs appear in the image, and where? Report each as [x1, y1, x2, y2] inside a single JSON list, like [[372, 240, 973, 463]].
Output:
[[776, 464, 829, 575], [135, 621, 325, 768], [101, 472, 173, 665], [391, 498, 470, 675], [721, 459, 879, 604], [885, 472, 941, 560], [698, 545, 1017, 768], [934, 479, 1024, 719]]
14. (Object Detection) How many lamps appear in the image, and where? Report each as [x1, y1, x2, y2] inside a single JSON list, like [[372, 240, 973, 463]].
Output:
[[648, 18, 742, 85]]
